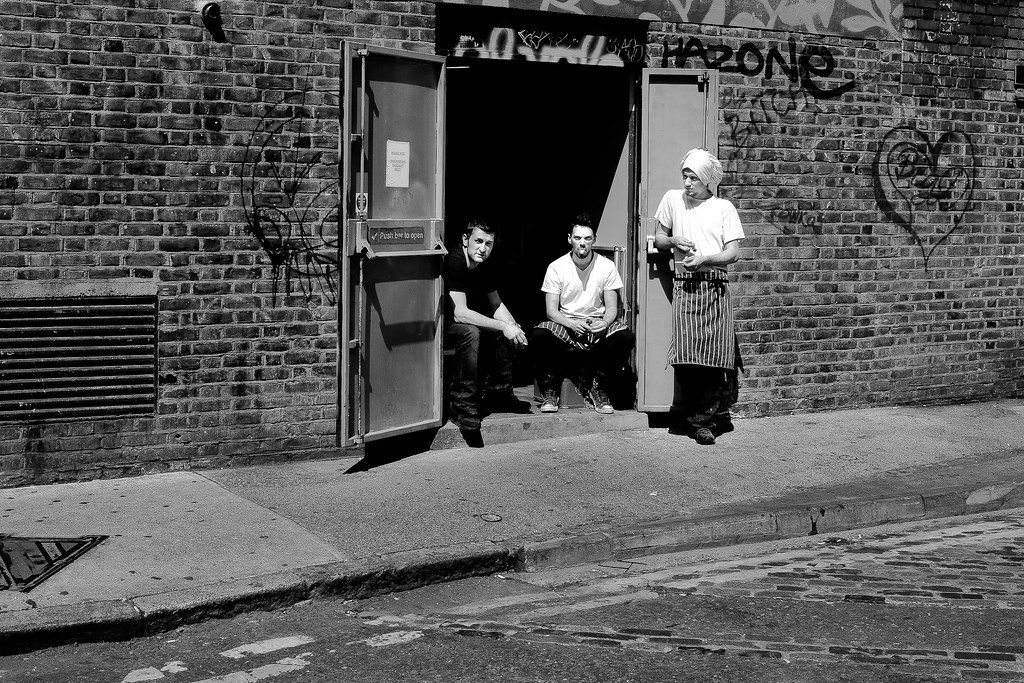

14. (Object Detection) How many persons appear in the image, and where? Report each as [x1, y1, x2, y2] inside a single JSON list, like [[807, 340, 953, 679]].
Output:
[[531, 214, 639, 415], [655, 148, 746, 444], [443, 218, 532, 433]]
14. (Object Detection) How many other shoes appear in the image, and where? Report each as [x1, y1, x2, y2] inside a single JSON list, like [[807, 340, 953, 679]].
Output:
[[488, 391, 534, 412], [675, 412, 716, 443], [448, 409, 481, 432]]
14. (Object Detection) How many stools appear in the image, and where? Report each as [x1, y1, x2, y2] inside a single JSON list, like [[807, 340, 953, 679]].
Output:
[[534, 377, 586, 411], [444, 347, 459, 357]]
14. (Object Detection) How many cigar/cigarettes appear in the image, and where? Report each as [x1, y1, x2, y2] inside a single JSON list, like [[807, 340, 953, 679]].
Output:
[[674, 261, 684, 264]]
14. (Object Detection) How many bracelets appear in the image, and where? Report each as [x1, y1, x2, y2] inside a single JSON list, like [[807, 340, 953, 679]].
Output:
[[510, 322, 519, 326], [601, 320, 609, 329]]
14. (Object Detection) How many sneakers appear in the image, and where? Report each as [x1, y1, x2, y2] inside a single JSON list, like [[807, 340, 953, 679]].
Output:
[[541, 391, 562, 412], [589, 389, 614, 413]]
